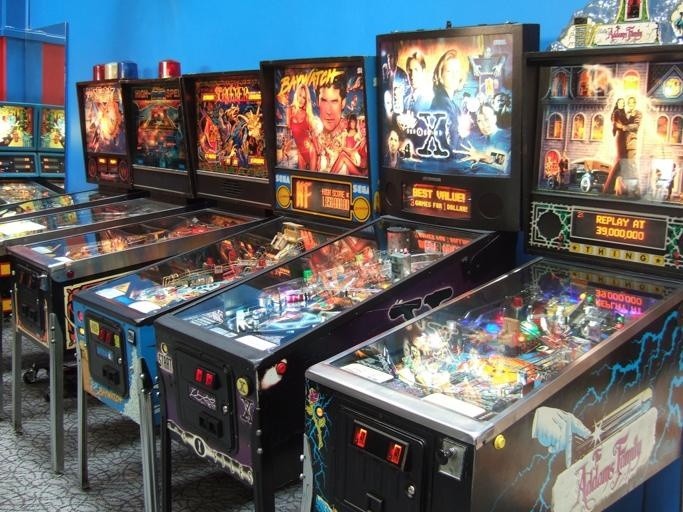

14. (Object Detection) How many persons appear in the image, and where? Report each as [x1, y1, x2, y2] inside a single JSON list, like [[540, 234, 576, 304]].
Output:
[[275, 72, 369, 178], [376, 41, 512, 178], [615, 96, 642, 200], [601, 98, 628, 197]]
